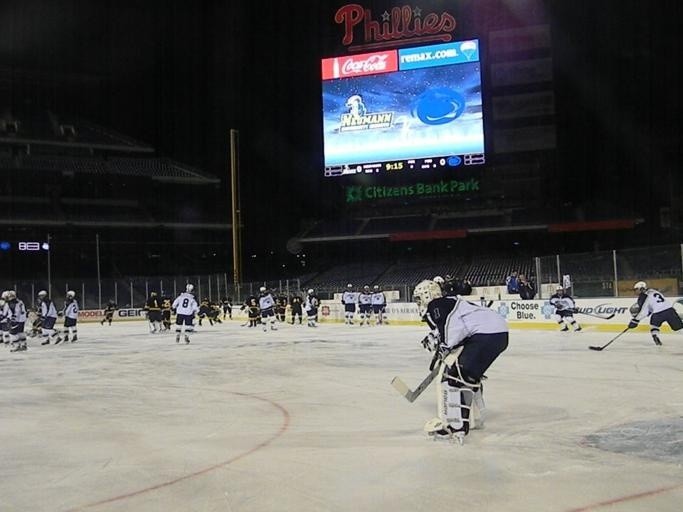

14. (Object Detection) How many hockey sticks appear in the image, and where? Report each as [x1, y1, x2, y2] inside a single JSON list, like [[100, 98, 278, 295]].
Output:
[[241, 312, 250, 327], [588, 328, 629, 350], [578, 312, 614, 320], [391, 361, 442, 403]]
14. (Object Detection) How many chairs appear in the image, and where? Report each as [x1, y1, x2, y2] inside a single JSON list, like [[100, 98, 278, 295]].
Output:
[[310, 209, 486, 237], [2, 152, 197, 178], [299, 256, 560, 292], [511, 206, 562, 225]]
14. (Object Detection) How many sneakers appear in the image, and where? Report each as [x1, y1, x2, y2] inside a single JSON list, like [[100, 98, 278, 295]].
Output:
[[0, 340, 27, 352], [345, 321, 383, 327], [560, 328, 584, 334], [435, 422, 472, 436], [175, 335, 190, 343], [39, 336, 77, 346], [246, 321, 319, 328], [149, 328, 171, 334], [263, 328, 277, 332]]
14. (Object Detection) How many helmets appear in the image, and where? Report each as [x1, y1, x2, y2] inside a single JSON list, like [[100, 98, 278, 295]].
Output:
[[0, 290, 17, 301], [556, 285, 563, 290], [413, 276, 444, 311], [38, 291, 47, 296], [259, 287, 266, 291], [347, 284, 353, 288], [634, 281, 647, 293], [66, 290, 75, 297], [307, 288, 314, 294], [185, 284, 194, 293], [364, 285, 379, 288], [150, 292, 158, 296]]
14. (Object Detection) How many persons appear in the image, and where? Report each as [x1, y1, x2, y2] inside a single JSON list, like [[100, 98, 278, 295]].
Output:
[[413, 278, 510, 440], [418, 273, 473, 354], [628, 280, 682, 346], [141, 282, 388, 344], [548, 285, 581, 332], [98, 298, 121, 326], [517, 273, 535, 300], [0, 287, 80, 354], [505, 269, 529, 300]]
